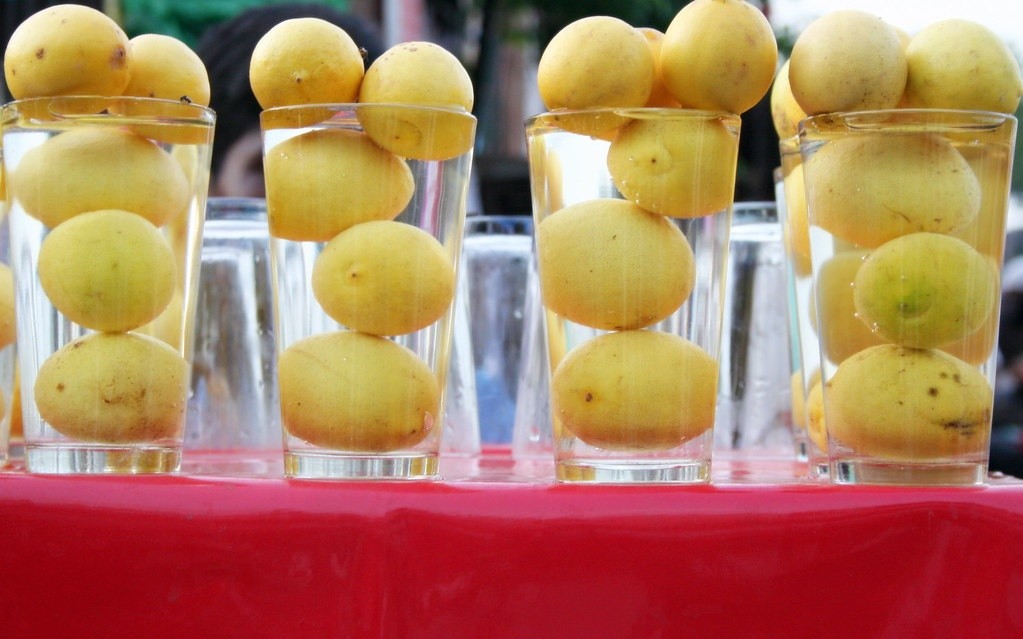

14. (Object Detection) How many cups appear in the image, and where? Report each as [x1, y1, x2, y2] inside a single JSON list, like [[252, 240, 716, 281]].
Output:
[[721, 106, 1019, 486], [0, 92, 216, 474], [260, 102, 478, 483], [519, 110, 741, 483], [428, 211, 558, 486], [178, 195, 289, 478]]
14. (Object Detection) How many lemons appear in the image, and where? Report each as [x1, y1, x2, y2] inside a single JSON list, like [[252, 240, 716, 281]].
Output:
[[0, 5, 211, 441], [772, 11, 1023, 457], [250, 17, 473, 451], [534, 0, 778, 452]]
